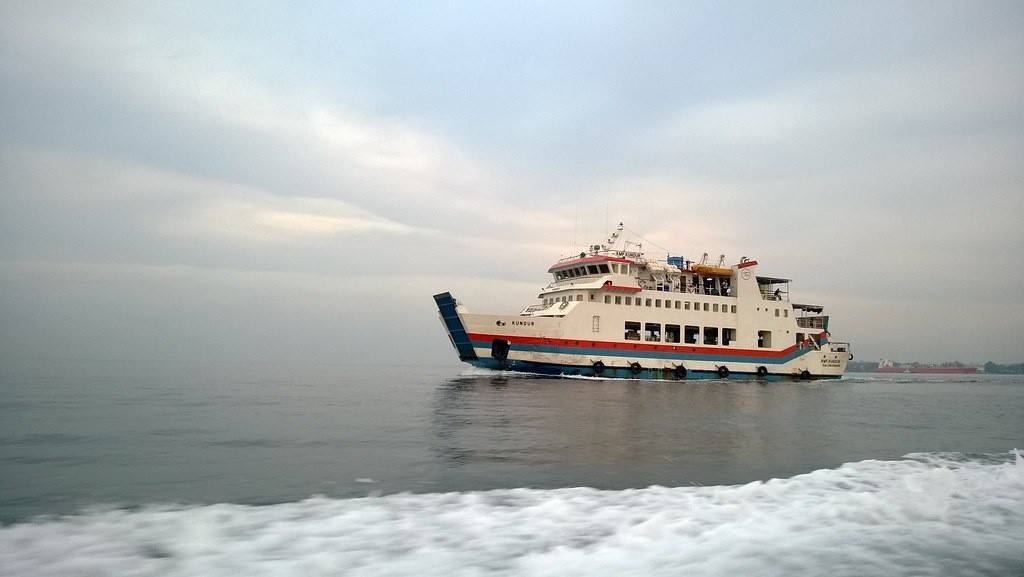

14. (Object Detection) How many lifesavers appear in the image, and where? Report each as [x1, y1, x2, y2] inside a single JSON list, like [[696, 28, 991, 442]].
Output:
[[758, 365, 768, 377], [718, 365, 730, 378], [801, 370, 811, 379], [675, 365, 687, 379], [630, 362, 642, 375], [592, 360, 605, 374], [848, 353, 854, 361]]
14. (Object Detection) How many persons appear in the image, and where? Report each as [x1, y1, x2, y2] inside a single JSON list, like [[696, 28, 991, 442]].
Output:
[[774, 288, 782, 300]]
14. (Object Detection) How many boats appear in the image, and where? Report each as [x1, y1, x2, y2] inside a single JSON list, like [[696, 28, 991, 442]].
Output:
[[430, 222, 850, 383]]
